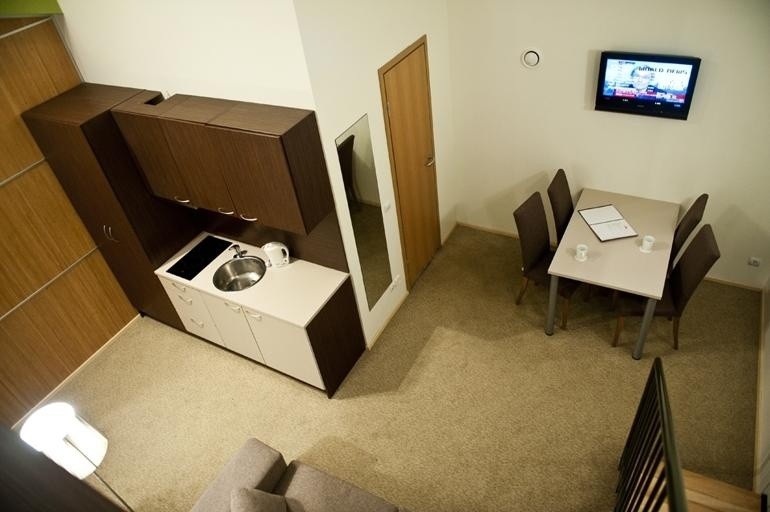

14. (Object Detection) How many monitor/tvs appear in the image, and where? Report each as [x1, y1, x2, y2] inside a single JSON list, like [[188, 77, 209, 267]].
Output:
[[595, 51, 701, 121]]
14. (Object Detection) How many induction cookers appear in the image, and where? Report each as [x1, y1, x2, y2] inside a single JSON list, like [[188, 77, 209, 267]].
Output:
[[165, 235, 233, 282]]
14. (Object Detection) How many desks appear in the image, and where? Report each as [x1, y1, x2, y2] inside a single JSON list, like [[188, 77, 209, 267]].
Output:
[[544, 187, 681, 360]]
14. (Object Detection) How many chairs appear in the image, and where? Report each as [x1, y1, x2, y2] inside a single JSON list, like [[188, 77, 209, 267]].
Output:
[[188, 439, 399, 512], [512, 170, 722, 348]]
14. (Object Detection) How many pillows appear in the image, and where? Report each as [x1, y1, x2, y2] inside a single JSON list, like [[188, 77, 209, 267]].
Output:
[[230, 482, 288, 512]]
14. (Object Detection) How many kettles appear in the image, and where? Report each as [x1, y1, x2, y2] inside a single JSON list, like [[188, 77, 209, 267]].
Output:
[[260, 241, 291, 268]]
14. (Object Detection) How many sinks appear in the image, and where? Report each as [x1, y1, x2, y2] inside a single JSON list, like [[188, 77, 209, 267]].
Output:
[[212, 255, 266, 292]]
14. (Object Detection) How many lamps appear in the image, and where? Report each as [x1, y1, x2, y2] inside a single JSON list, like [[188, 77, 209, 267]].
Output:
[[19, 401, 137, 512]]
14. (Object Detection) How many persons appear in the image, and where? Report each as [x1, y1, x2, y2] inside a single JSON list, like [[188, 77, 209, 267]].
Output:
[[604, 64, 667, 102]]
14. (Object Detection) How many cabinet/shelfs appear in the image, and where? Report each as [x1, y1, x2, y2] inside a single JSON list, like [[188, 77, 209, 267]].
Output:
[[110, 90, 335, 237], [158, 277, 366, 398], [18, 82, 188, 338]]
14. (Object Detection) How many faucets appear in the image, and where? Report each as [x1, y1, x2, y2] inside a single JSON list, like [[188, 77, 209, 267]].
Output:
[[227, 244, 247, 258]]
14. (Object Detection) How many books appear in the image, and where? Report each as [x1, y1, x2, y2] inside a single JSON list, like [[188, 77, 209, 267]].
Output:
[[578, 204, 639, 242]]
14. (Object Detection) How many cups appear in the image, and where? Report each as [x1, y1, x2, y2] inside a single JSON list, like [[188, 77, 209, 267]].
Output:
[[642, 235, 655, 250], [577, 245, 588, 260]]
